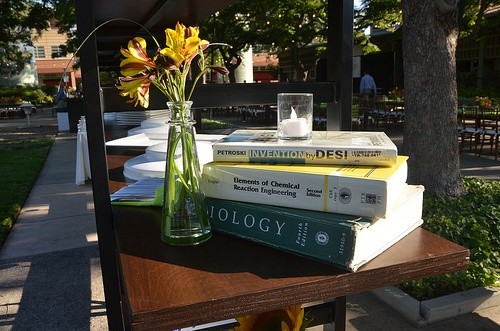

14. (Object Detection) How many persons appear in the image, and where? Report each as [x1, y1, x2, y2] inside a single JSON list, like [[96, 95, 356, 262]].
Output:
[[56, 83, 67, 102], [360, 71, 376, 96], [78, 82, 82, 91]]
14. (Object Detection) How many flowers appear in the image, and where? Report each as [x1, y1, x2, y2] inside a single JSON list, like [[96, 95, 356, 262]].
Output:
[[60, 17, 232, 234], [476, 96, 496, 106]]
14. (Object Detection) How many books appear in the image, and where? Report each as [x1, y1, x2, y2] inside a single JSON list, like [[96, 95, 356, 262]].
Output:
[[205, 185, 427, 272], [212, 129, 398, 167], [203, 155, 409, 220]]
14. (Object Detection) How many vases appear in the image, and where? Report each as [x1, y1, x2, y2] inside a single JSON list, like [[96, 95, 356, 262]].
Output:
[[163, 100, 213, 245]]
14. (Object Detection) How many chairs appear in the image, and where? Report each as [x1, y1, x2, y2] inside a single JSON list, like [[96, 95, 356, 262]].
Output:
[[234, 104, 500, 162]]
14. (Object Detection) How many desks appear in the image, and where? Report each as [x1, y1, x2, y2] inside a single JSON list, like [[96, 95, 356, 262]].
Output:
[[457, 113, 500, 160]]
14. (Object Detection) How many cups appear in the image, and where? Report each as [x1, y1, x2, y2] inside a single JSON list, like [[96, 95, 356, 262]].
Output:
[[277, 93, 314, 140]]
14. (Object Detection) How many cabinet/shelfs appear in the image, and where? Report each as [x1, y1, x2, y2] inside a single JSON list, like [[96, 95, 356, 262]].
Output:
[[79, 0, 471, 331]]
